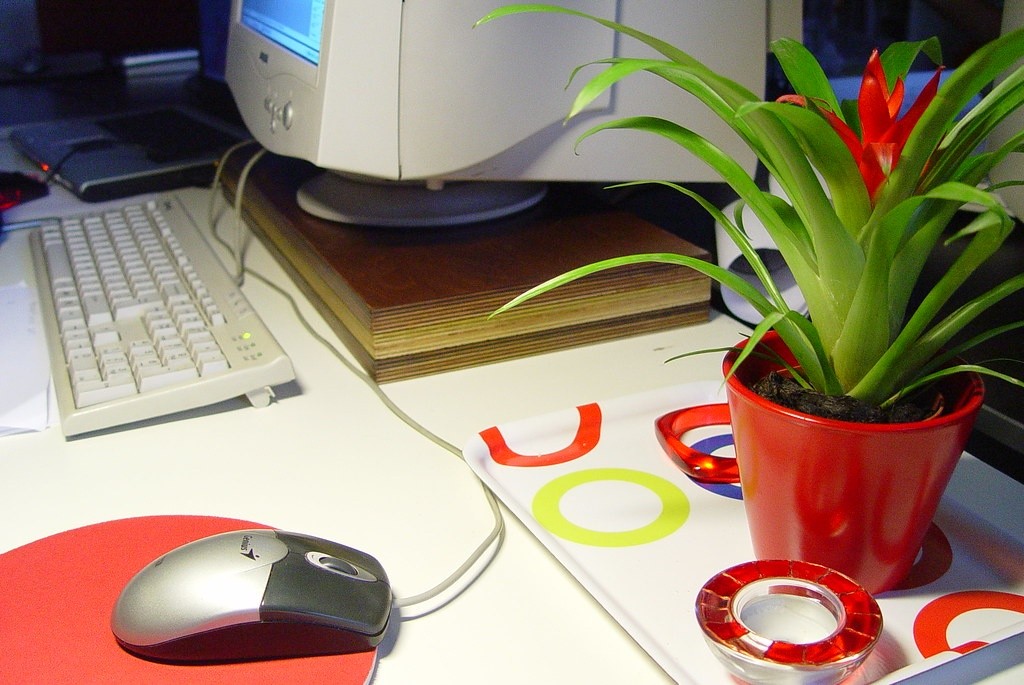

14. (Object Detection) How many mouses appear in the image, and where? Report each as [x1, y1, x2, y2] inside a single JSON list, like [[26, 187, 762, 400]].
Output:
[[109, 528, 393, 659]]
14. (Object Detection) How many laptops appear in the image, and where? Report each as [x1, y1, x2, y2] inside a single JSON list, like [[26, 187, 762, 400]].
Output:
[[11, 0, 252, 203]]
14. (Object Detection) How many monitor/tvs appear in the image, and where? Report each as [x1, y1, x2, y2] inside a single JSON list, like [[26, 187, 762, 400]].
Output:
[[223, 1, 765, 230]]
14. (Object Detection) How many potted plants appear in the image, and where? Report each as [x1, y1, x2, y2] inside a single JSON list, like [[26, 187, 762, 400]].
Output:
[[468, 6, 1024, 601]]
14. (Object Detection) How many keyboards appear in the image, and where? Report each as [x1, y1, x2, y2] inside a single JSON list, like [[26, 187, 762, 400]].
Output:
[[27, 192, 297, 437]]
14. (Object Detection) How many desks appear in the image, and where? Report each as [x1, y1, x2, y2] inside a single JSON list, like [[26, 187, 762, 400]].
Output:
[[0, 48, 1024, 685]]
